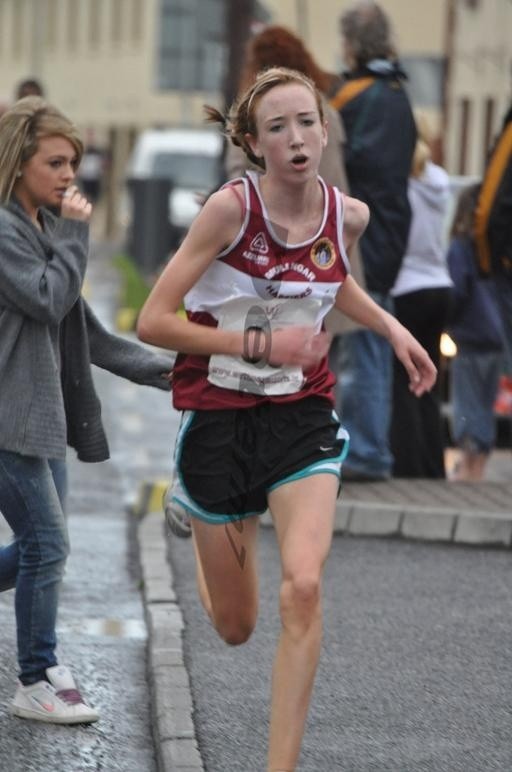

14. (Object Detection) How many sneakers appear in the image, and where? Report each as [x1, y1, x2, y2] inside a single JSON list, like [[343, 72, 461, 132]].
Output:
[[11, 662, 106, 729]]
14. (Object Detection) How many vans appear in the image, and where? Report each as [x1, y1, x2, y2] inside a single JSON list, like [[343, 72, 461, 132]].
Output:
[[123, 125, 231, 241]]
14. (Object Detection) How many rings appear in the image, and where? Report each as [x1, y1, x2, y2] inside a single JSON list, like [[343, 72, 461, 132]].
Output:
[[62, 193, 73, 198]]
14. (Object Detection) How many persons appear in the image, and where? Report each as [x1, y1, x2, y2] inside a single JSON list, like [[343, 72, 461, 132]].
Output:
[[76, 143, 105, 205], [1, 95, 185, 731], [132, 62, 442, 770]]
